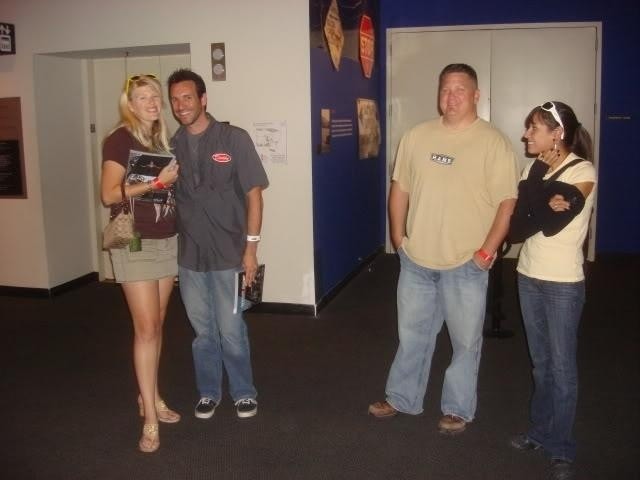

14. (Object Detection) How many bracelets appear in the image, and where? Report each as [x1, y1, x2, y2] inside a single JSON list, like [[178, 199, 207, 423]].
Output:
[[478, 249, 494, 262], [245, 234, 261, 241], [147, 181, 153, 192], [153, 177, 163, 190]]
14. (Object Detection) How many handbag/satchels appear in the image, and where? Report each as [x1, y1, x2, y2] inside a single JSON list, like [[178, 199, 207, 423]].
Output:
[[102, 211, 134, 250]]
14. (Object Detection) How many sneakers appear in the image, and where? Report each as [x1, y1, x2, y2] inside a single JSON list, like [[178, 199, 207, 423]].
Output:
[[548, 456, 573, 479], [437, 414, 466, 435], [194, 398, 217, 419], [510, 432, 545, 456], [235, 399, 259, 419], [368, 400, 399, 418]]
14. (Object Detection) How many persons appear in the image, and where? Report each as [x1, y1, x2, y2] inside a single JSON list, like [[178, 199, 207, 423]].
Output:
[[506, 101, 598, 480], [161, 68, 269, 421], [363, 62, 521, 435], [97, 72, 185, 454]]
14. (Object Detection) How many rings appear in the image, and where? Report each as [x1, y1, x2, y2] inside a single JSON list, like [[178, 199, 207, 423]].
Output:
[[542, 159, 545, 162], [555, 206, 557, 208]]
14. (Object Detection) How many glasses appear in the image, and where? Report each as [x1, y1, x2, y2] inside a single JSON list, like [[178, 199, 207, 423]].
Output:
[[541, 100, 565, 139], [126, 74, 156, 94]]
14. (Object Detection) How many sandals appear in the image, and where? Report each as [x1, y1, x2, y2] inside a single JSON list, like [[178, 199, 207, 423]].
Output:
[[136, 399, 182, 424], [139, 424, 159, 453]]
